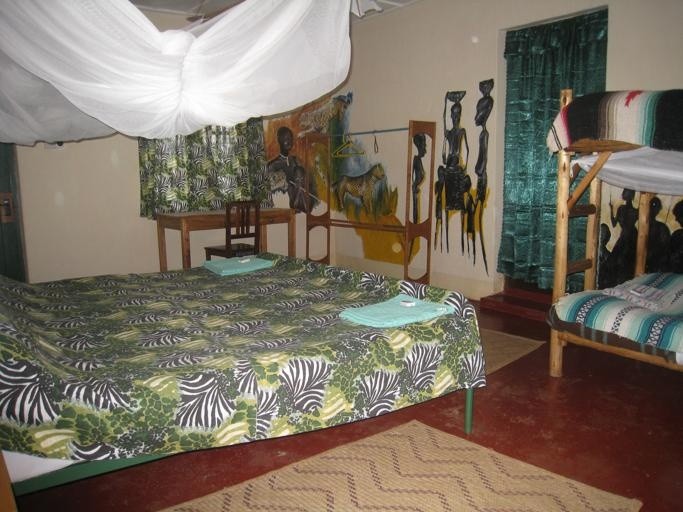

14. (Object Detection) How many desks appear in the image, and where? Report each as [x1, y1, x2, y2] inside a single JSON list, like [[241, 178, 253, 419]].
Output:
[[156, 207, 296, 271]]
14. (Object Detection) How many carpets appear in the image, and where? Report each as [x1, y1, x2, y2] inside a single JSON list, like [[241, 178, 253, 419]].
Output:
[[165, 418, 643, 512], [478, 325, 548, 376]]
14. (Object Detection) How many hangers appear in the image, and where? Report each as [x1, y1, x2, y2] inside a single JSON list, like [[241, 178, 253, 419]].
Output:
[[304, 120, 435, 286], [372, 130, 378, 153], [330, 129, 363, 158], [335, 130, 365, 158]]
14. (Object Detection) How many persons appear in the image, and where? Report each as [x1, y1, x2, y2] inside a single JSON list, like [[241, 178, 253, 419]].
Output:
[[434, 166, 443, 254], [268, 127, 316, 214], [608, 187, 638, 285], [472, 79, 494, 277], [442, 91, 469, 256], [412, 133, 427, 225], [599, 223, 612, 275], [670, 200, 683, 273], [644, 196, 671, 274], [464, 175, 475, 258]]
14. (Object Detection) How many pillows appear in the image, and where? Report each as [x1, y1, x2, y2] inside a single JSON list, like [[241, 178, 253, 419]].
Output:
[[601, 280, 675, 311]]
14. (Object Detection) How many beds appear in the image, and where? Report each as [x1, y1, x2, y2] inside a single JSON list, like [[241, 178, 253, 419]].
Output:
[[546, 89, 683, 377], [0, 252, 486, 511]]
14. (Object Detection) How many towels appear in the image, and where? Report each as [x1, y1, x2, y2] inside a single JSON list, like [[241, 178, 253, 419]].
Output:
[[204, 256, 273, 276], [340, 294, 453, 330]]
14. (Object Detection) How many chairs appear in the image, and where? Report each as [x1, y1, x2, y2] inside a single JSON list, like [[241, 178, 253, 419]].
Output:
[[203, 199, 259, 260]]
